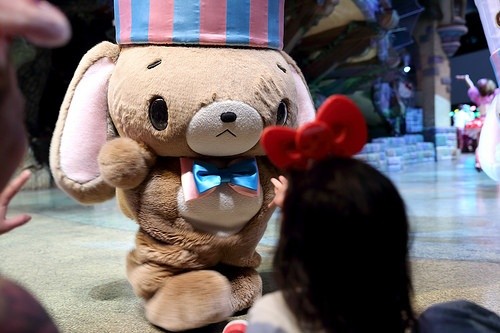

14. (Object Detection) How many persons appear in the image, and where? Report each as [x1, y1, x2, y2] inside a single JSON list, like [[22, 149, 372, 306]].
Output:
[[246, 72, 500, 333], [0, 0, 73, 333]]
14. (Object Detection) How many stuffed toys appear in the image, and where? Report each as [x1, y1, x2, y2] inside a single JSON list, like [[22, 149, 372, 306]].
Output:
[[49, 0, 319, 332]]
